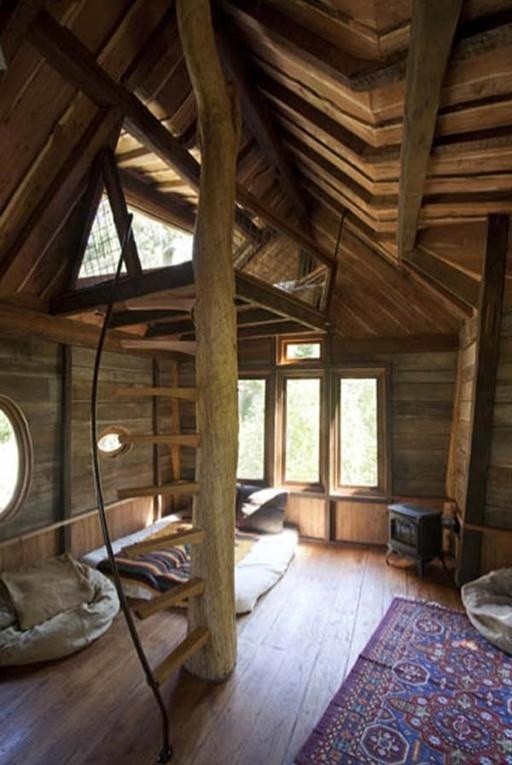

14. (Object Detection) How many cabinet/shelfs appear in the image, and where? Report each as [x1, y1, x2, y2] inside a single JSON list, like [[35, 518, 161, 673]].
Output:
[[385, 503, 450, 581]]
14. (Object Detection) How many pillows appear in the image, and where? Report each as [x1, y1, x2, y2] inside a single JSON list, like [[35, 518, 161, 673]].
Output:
[[235, 482, 289, 534]]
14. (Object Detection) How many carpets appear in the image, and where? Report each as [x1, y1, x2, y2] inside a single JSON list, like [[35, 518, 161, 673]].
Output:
[[292, 597, 512, 764]]
